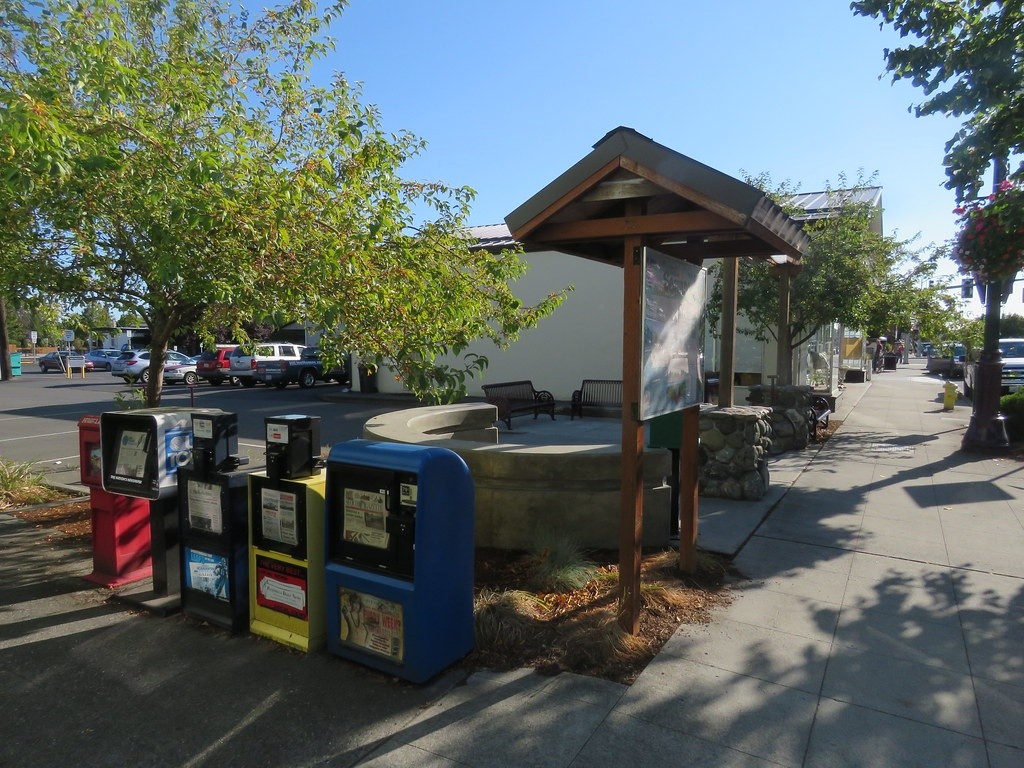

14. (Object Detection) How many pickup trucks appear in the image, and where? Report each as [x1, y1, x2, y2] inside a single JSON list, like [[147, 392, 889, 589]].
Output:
[[252, 346, 365, 389]]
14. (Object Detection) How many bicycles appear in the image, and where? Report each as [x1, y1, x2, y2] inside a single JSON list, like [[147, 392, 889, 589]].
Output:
[[706, 377, 797, 456]]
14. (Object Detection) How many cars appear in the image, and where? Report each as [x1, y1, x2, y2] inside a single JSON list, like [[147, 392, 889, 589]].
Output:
[[163, 354, 208, 386], [921, 341, 968, 380], [83, 348, 122, 372], [37, 350, 94, 374]]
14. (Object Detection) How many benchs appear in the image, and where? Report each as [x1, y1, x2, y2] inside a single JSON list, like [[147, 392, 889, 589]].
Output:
[[570, 379, 624, 421], [481, 380, 555, 429], [801, 390, 832, 440]]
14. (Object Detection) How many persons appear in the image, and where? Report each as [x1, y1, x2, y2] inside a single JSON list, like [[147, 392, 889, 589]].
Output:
[[876, 340, 883, 354], [898, 342, 905, 353]]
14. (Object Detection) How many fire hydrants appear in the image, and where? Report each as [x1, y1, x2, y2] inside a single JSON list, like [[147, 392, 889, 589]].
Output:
[[942, 378, 959, 413]]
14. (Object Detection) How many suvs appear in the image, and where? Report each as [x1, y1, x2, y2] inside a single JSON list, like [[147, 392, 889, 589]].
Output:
[[225, 342, 307, 388], [111, 347, 191, 384], [959, 337, 1024, 402], [196, 343, 250, 387]]
